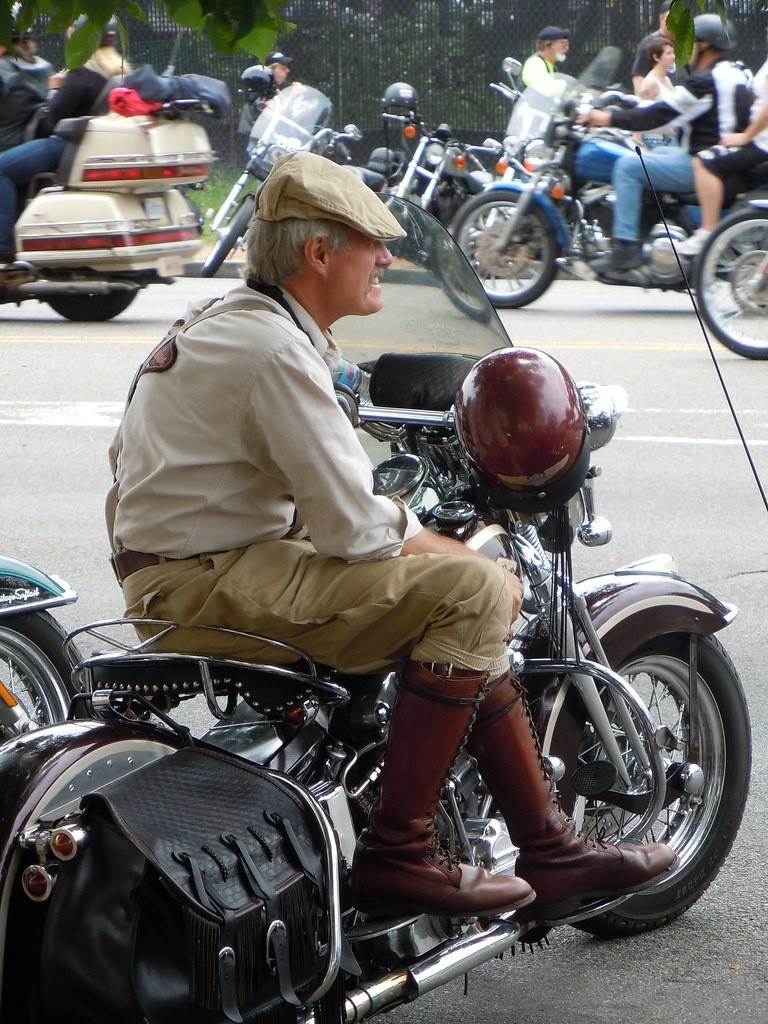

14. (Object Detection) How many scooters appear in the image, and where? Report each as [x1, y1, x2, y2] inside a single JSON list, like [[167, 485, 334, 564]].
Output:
[[694, 196, 768, 361], [0, 551, 96, 742], [0, 94, 216, 324]]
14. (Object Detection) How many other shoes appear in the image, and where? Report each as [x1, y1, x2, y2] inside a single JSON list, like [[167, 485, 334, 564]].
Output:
[[674, 230, 712, 255], [589, 239, 643, 272]]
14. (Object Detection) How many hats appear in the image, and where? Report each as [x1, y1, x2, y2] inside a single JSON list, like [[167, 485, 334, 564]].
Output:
[[538, 26, 568, 39], [255, 152, 407, 241], [265, 51, 293, 65]]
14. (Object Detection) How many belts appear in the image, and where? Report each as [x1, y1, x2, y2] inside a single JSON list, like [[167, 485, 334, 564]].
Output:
[[111, 550, 177, 584]]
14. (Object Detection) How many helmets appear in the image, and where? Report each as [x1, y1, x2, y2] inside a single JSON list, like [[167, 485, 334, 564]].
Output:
[[381, 82, 419, 110], [13, 25, 33, 38], [73, 12, 121, 43], [693, 13, 736, 51], [240, 64, 274, 89], [454, 347, 588, 512]]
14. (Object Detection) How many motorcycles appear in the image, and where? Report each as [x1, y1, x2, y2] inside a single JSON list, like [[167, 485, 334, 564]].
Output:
[[362, 58, 552, 281], [447, 46, 744, 311], [0, 189, 754, 1024], [200, 66, 478, 280]]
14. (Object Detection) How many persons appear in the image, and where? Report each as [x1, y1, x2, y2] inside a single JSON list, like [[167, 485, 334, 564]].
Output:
[[238, 51, 301, 135], [516, 26, 591, 113], [104, 151, 677, 925], [575, 0, 768, 273], [0, 0, 133, 263]]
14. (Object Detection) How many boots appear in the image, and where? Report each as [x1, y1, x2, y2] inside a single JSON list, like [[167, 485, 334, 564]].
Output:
[[351, 659, 537, 918], [464, 666, 680, 920]]
